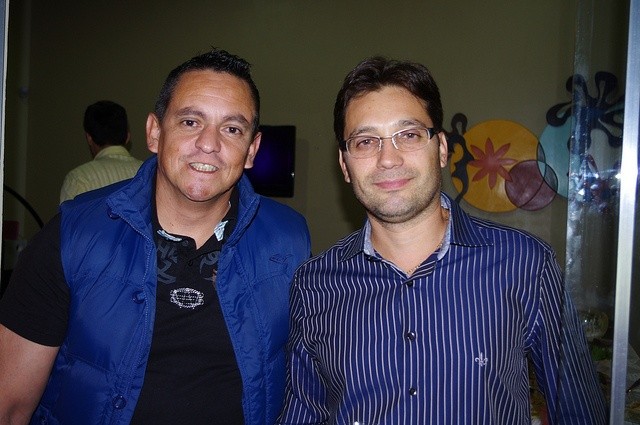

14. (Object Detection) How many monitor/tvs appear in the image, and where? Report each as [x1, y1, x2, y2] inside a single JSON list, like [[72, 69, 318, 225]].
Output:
[[243, 125, 296, 198]]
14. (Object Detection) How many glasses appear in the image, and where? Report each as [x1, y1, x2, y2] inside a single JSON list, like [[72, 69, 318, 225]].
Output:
[[340, 124, 439, 159]]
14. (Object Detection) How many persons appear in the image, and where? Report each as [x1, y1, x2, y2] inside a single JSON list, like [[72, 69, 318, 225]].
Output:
[[58, 99, 144, 208], [0, 48, 313, 425], [274, 52, 612, 425]]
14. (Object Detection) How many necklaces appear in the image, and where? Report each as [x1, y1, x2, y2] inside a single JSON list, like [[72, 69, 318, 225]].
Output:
[[406, 205, 449, 277]]
